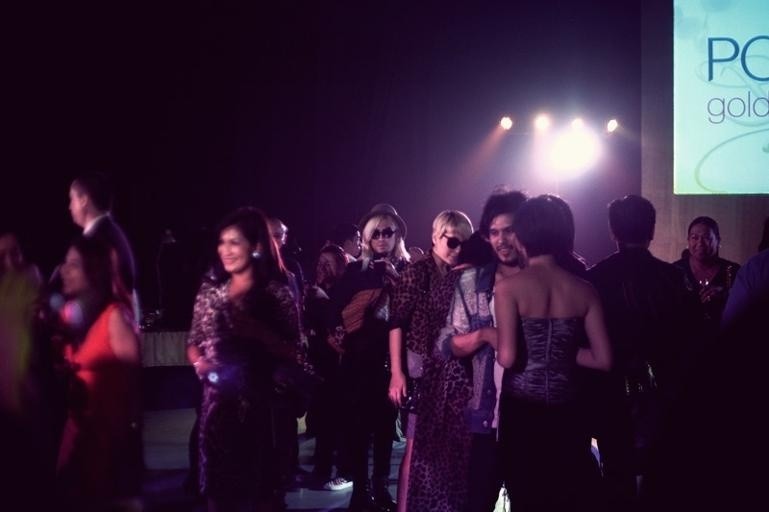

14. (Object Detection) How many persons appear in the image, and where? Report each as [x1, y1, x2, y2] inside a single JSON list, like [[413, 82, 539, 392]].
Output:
[[0, 170, 767, 512]]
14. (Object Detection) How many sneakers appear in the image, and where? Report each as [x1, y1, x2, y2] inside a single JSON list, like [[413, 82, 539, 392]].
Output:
[[324, 478, 353, 491]]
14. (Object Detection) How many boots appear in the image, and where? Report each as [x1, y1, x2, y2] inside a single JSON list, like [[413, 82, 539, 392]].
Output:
[[349, 479, 396, 511]]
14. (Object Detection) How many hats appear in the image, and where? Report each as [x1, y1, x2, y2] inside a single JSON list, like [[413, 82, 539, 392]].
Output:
[[358, 204, 407, 238]]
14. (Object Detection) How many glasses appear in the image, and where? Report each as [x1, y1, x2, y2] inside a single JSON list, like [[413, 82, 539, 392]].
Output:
[[372, 227, 396, 240], [443, 234, 467, 250]]
